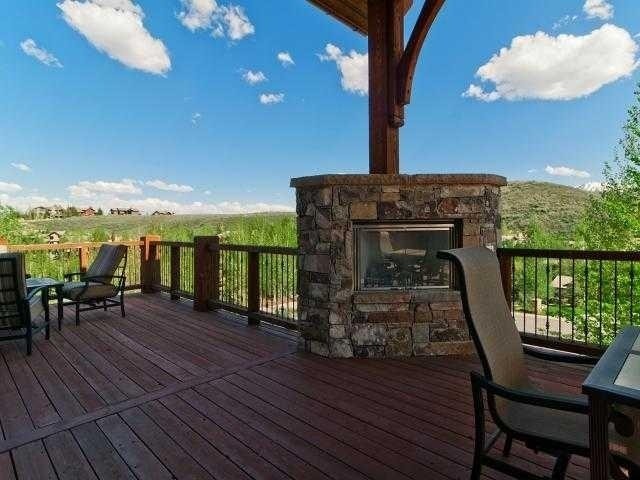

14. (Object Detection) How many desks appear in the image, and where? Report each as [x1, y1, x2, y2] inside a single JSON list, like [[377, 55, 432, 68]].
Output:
[[580, 323, 640, 480]]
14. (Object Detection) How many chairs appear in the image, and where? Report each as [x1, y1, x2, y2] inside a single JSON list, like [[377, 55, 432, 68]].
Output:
[[0, 244, 129, 356], [435, 245, 639, 480]]
